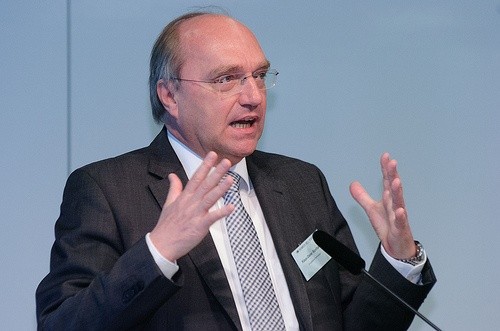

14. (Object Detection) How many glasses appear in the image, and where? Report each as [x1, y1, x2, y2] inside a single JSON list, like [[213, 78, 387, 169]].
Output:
[[160, 69, 279, 99]]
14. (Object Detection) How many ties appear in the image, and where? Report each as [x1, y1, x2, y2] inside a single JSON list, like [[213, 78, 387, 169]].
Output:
[[218, 171, 287, 331]]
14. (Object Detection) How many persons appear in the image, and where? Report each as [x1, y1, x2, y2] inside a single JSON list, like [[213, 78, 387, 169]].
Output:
[[35, 12, 437, 331]]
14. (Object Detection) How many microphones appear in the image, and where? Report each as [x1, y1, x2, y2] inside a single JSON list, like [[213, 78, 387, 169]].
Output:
[[312, 230, 441, 331]]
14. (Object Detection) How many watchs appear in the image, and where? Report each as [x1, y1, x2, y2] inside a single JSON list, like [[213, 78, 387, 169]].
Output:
[[396, 240, 424, 267]]
